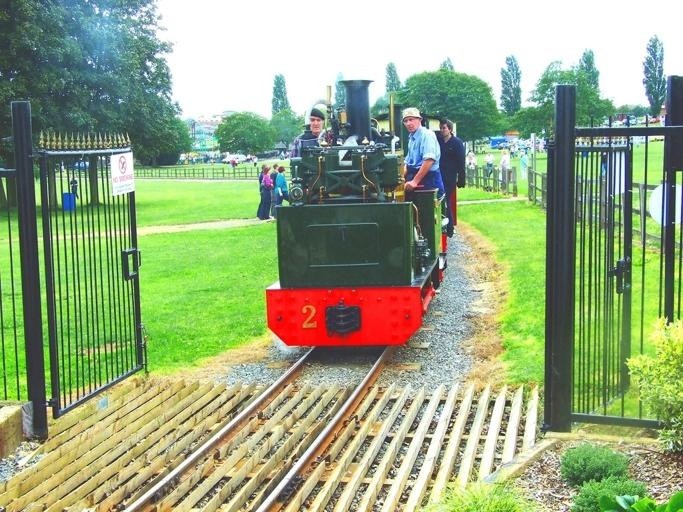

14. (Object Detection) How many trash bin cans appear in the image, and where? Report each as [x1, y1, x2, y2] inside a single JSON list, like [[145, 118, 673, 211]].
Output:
[[63, 192, 74, 209], [583, 151, 588, 156]]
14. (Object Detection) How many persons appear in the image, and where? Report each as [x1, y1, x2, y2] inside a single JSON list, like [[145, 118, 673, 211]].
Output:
[[433, 117, 466, 239], [273, 166, 290, 217], [269, 164, 278, 216], [68, 176, 79, 200], [259, 168, 272, 220], [400, 107, 444, 242], [464, 135, 546, 183], [171, 148, 291, 168], [291, 107, 324, 180], [256, 165, 266, 218]]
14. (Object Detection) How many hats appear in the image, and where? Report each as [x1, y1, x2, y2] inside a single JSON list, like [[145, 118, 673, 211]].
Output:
[[311, 108, 325, 120], [400, 107, 424, 123], [263, 166, 270, 175], [278, 167, 285, 172]]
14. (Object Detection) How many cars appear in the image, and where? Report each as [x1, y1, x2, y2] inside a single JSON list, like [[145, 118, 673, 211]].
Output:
[[74, 158, 88, 171], [623, 112, 659, 125], [490, 137, 544, 150], [220, 152, 247, 165]]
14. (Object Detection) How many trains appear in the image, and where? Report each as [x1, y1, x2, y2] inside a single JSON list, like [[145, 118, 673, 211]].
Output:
[[265, 79, 459, 349]]
